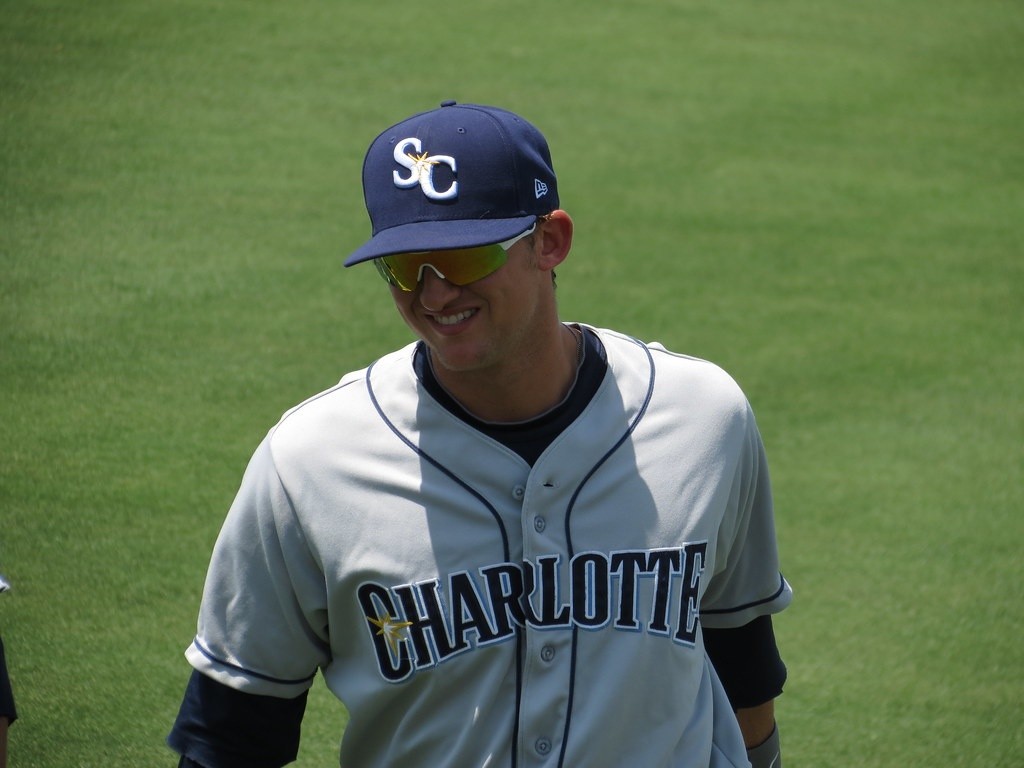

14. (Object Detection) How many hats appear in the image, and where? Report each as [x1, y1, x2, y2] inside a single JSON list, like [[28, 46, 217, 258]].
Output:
[[344, 100, 559, 268]]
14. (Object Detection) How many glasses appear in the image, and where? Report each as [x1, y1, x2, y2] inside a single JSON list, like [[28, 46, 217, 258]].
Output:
[[374, 222, 536, 291]]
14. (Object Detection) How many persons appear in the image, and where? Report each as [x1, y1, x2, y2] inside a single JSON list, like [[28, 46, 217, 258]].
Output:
[[166, 100, 793, 768]]
